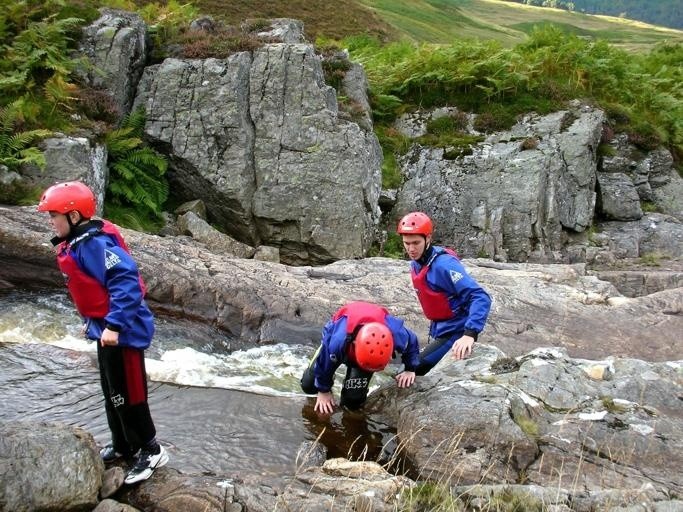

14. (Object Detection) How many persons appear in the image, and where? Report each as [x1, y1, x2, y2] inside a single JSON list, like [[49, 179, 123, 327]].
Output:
[[38, 181, 169, 485], [300, 301, 420, 414], [397, 212, 492, 376]]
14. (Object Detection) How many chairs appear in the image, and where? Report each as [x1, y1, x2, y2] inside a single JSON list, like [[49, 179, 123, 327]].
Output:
[[124, 445, 170, 485], [100, 440, 141, 463]]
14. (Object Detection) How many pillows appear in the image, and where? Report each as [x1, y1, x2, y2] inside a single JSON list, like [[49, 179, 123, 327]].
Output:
[[37, 180, 97, 219], [355, 321, 394, 371], [397, 211, 433, 236]]
